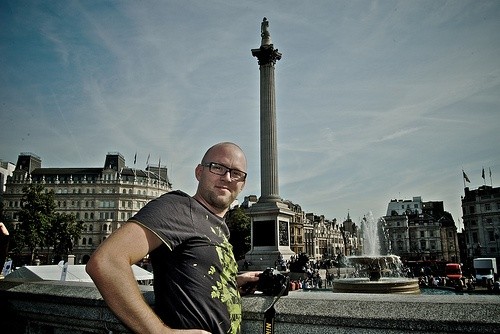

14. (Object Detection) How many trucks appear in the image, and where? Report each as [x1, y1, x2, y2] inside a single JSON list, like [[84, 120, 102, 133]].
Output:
[[445, 263, 463, 287], [474, 257, 498, 287]]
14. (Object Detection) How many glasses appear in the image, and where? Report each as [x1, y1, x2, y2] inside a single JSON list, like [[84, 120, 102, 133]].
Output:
[[201, 161, 247, 183]]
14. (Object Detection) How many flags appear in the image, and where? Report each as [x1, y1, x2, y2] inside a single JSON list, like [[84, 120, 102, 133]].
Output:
[[132, 151, 161, 168], [462, 170, 471, 183], [481, 168, 492, 180]]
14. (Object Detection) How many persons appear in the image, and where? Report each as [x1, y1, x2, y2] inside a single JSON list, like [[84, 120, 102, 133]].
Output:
[[285, 251, 500, 296], [83, 142, 267, 334], [0, 221, 11, 276]]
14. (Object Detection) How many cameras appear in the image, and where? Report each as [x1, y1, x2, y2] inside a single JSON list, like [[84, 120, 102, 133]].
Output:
[[257, 268, 287, 291]]
